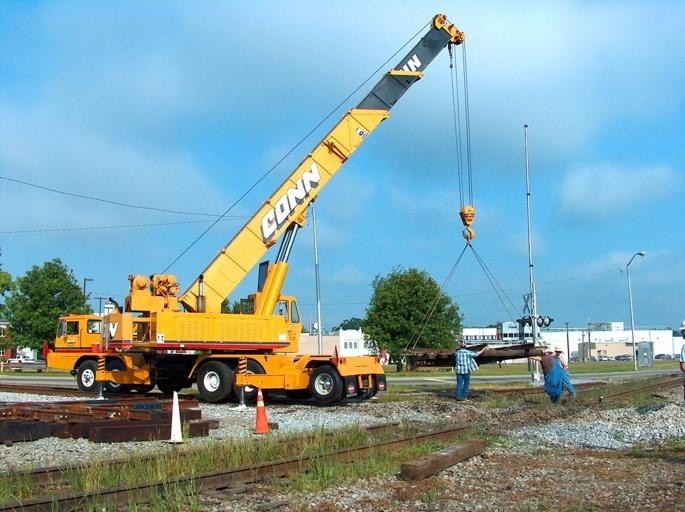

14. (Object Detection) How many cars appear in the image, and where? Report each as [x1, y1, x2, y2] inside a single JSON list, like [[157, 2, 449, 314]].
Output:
[[6, 354, 35, 366], [590, 354, 681, 361]]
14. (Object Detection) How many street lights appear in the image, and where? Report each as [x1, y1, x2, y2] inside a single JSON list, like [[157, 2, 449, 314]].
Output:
[[82, 278, 93, 308], [626, 251, 646, 372], [587, 318, 593, 362]]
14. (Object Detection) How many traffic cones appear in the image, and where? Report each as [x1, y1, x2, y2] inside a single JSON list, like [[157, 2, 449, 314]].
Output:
[[164, 391, 188, 445], [252, 386, 272, 434]]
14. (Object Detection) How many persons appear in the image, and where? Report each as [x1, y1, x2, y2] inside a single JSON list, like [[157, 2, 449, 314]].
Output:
[[452, 341, 489, 402], [554, 346, 577, 399], [528, 348, 563, 404], [679, 320, 685, 402]]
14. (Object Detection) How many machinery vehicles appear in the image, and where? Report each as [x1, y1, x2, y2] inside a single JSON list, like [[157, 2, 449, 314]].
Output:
[[42, 12, 478, 407]]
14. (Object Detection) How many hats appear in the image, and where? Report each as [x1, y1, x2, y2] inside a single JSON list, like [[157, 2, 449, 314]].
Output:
[[542, 348, 553, 353], [554, 346, 563, 352]]
